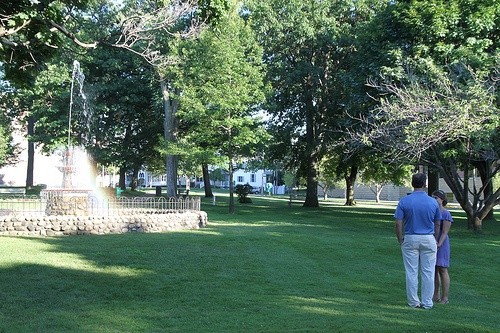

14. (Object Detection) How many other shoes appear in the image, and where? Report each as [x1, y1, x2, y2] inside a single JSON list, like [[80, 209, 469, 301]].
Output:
[[433, 297, 441, 304], [439, 299, 450, 306]]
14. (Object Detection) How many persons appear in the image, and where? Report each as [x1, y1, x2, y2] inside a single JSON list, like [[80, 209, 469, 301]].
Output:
[[432, 190, 454, 305], [394, 174, 442, 310]]
